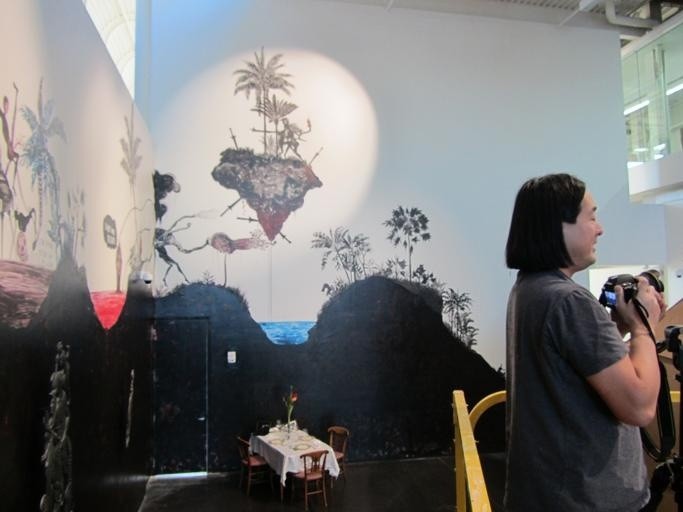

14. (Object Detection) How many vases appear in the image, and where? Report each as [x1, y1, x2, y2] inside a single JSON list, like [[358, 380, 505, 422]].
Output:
[[287, 424, 291, 440]]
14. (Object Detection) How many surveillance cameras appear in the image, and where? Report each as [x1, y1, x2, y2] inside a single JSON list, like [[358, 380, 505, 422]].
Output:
[[144, 274, 152, 284]]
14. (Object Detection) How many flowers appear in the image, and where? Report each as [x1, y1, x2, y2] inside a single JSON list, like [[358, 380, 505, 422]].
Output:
[[282, 385, 298, 425]]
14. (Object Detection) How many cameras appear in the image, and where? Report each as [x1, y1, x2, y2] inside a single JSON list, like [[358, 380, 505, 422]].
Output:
[[602, 269, 665, 309]]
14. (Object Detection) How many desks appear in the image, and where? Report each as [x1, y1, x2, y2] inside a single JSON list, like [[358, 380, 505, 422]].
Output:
[[248, 425, 341, 500]]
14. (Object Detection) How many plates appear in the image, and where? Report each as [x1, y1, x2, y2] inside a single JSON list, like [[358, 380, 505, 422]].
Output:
[[272, 435, 313, 450]]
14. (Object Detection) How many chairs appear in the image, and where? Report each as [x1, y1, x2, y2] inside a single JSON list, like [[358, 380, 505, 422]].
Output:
[[286, 451, 329, 512], [328, 426, 350, 488], [234, 435, 275, 497]]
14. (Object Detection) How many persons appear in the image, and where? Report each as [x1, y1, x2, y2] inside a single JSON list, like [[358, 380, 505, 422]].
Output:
[[500, 172, 670, 512]]
